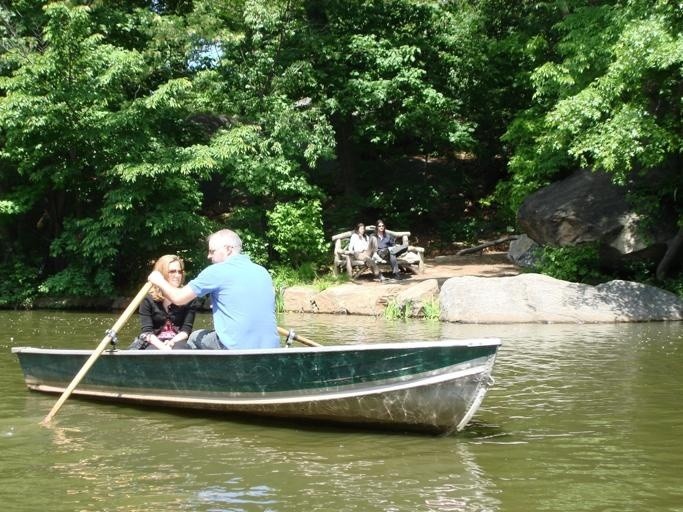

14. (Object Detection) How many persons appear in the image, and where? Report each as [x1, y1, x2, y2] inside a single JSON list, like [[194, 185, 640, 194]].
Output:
[[348, 223, 388, 286], [133, 250, 198, 350], [145, 229, 281, 351], [372, 219, 410, 276]]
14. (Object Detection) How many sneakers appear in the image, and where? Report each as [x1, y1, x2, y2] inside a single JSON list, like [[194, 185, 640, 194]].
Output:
[[394, 273, 401, 280], [372, 274, 389, 282], [374, 257, 387, 264]]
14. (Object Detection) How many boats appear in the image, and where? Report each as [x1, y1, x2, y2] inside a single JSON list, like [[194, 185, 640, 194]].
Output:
[[10, 332, 503, 440]]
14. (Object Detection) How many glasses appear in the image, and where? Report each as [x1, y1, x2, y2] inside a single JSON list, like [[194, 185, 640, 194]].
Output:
[[167, 269, 182, 274]]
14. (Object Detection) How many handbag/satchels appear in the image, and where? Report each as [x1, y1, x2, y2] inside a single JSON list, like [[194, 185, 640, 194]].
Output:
[[377, 248, 390, 261]]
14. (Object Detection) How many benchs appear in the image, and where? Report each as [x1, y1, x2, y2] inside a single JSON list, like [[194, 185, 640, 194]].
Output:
[[330, 225, 424, 282]]
